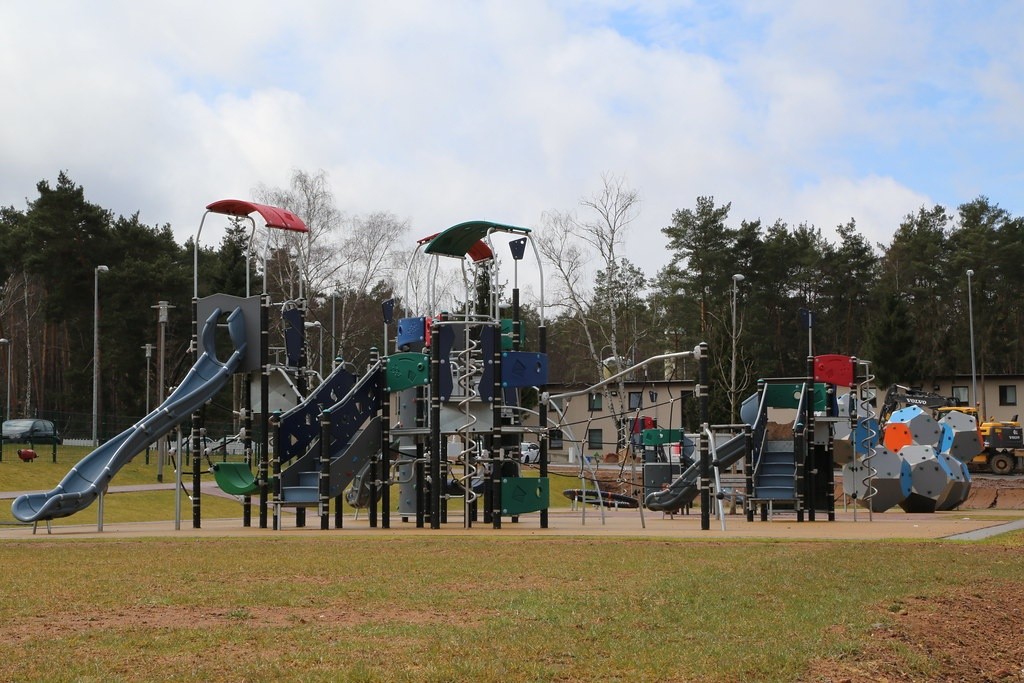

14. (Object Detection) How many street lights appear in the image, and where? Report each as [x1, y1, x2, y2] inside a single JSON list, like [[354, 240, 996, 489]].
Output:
[[92, 265, 109, 445], [731, 272, 745, 432], [967, 269, 979, 410]]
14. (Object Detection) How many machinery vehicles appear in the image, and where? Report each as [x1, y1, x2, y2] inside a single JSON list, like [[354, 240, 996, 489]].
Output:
[[878, 383, 1023, 476]]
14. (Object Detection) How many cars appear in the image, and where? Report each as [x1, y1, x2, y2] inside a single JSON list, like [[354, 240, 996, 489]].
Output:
[[2, 418, 64, 445], [511, 442, 540, 463], [176, 436, 260, 454]]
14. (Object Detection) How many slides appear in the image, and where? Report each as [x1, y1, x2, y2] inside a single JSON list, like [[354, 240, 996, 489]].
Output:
[[10, 352, 246, 525], [645, 433, 746, 510]]
